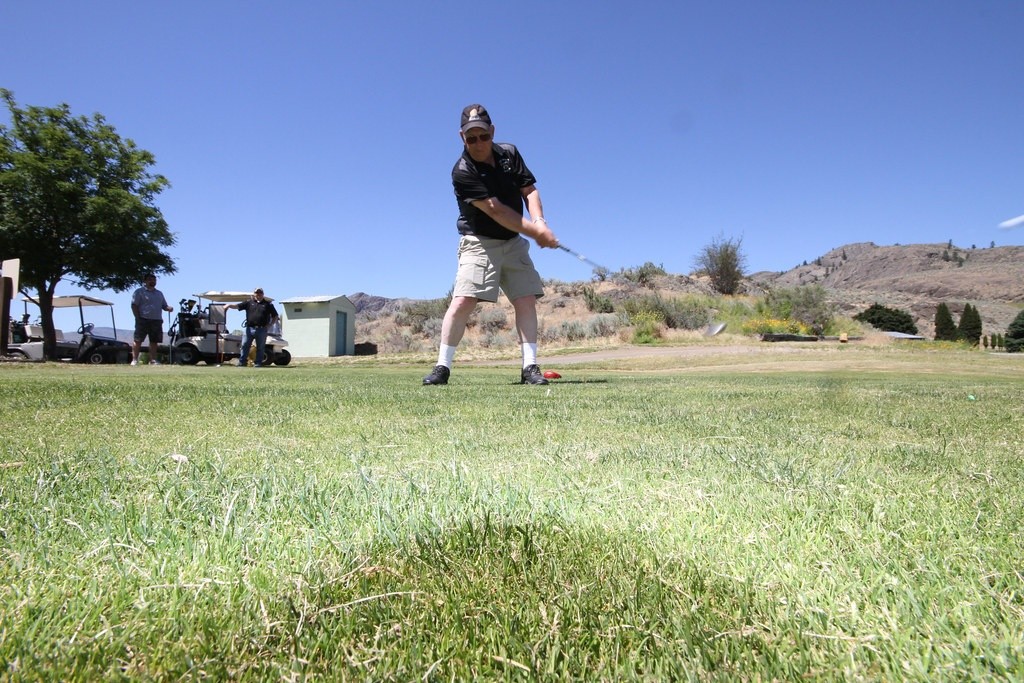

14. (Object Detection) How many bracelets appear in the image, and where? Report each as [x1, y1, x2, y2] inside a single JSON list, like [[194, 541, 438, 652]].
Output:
[[532, 217, 546, 224]]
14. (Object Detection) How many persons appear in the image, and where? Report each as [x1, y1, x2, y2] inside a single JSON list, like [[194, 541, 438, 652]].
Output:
[[423, 104, 556, 384], [131, 274, 173, 365], [223, 288, 278, 368]]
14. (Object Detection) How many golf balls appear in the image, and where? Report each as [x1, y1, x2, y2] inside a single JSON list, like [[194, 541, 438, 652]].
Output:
[[544, 370, 562, 379]]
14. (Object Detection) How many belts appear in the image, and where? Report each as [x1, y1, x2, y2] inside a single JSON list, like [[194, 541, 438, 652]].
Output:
[[247, 325, 265, 328], [458, 228, 493, 236]]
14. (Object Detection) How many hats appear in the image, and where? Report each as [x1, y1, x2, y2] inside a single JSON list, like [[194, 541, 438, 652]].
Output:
[[254, 287, 264, 294], [144, 274, 157, 278], [461, 103, 492, 133]]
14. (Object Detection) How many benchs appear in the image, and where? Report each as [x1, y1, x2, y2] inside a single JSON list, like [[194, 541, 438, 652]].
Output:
[[24, 325, 68, 344], [199, 319, 242, 341]]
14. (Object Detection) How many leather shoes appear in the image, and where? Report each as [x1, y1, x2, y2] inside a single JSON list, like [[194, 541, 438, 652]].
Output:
[[522, 364, 548, 384], [423, 365, 450, 384]]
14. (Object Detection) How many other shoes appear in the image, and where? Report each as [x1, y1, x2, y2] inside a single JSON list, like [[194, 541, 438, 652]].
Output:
[[236, 363, 247, 367], [131, 360, 137, 365], [148, 359, 161, 366], [254, 363, 261, 368]]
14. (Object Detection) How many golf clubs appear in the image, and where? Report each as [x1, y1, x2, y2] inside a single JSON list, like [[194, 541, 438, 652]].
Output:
[[556, 244, 726, 336]]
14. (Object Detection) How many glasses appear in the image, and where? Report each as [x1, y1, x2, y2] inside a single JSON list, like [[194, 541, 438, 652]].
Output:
[[466, 132, 491, 144], [255, 291, 263, 293]]
